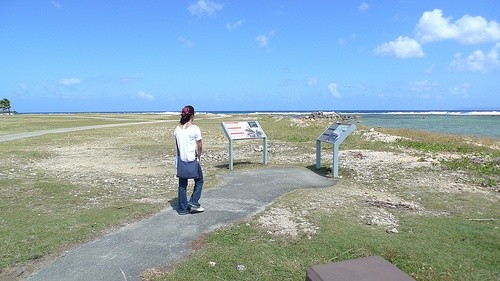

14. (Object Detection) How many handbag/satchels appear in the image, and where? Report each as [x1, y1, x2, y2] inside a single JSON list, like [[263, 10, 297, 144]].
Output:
[[176, 157, 200, 178]]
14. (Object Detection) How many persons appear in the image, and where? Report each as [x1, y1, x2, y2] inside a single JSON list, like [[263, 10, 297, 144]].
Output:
[[173, 105, 204, 215]]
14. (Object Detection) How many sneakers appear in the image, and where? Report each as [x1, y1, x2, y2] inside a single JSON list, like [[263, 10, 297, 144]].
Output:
[[190, 206, 204, 211], [180, 208, 190, 215]]
